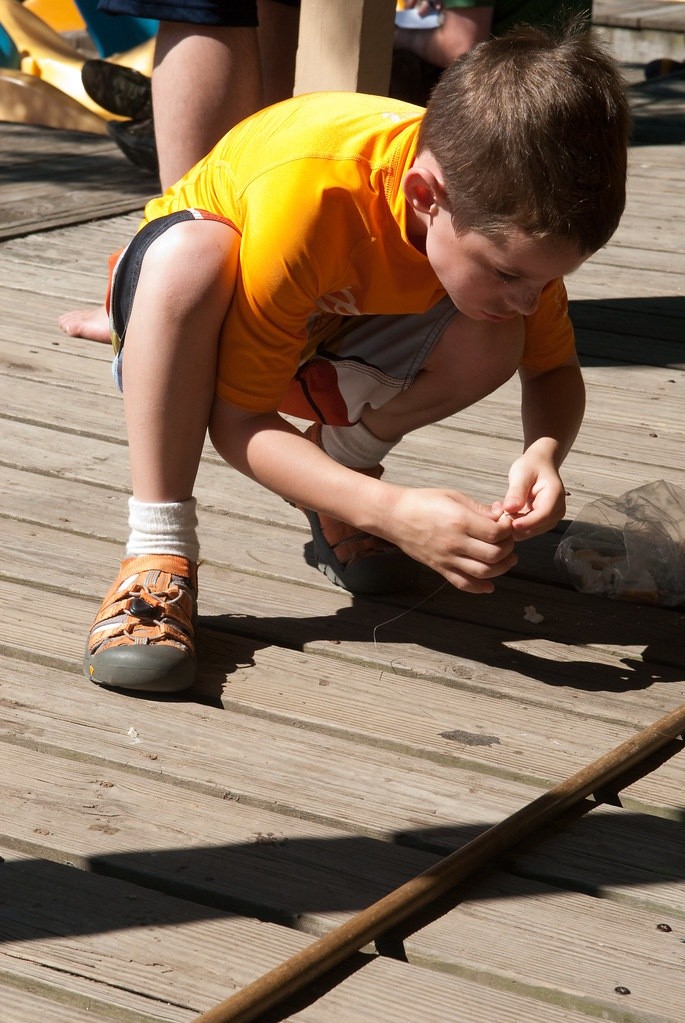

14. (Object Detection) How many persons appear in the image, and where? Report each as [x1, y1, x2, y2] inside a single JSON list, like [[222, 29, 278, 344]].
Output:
[[58, 0, 300, 344], [82, 30, 628, 692], [82, 0, 593, 175]]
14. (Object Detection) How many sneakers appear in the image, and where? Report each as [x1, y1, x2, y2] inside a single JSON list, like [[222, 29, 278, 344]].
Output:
[[82, 60, 152, 118], [84, 554, 204, 691], [107, 120, 160, 177], [281, 422, 416, 594]]
